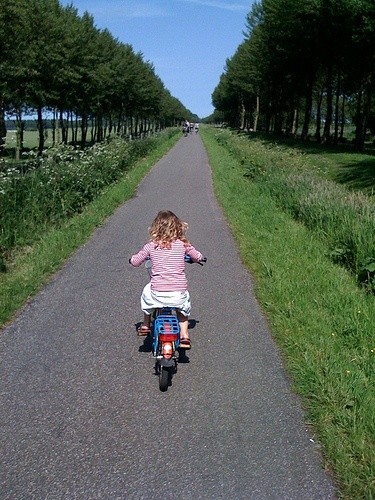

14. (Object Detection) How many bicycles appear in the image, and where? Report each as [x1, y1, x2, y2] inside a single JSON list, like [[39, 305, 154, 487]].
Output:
[[194, 126, 199, 135], [190, 125, 193, 135], [128, 254, 207, 392]]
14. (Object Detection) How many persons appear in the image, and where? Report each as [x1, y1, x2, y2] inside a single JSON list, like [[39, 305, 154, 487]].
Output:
[[130, 209, 203, 350], [182, 119, 199, 134]]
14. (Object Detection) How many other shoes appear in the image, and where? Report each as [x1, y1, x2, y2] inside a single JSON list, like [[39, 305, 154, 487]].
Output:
[[179, 334, 192, 349], [136, 323, 152, 336]]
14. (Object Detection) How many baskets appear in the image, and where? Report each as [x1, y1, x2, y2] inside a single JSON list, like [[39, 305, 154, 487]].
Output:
[[145, 260, 152, 276]]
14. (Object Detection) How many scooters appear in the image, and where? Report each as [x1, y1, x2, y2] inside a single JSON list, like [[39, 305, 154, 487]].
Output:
[[182, 123, 189, 137]]
